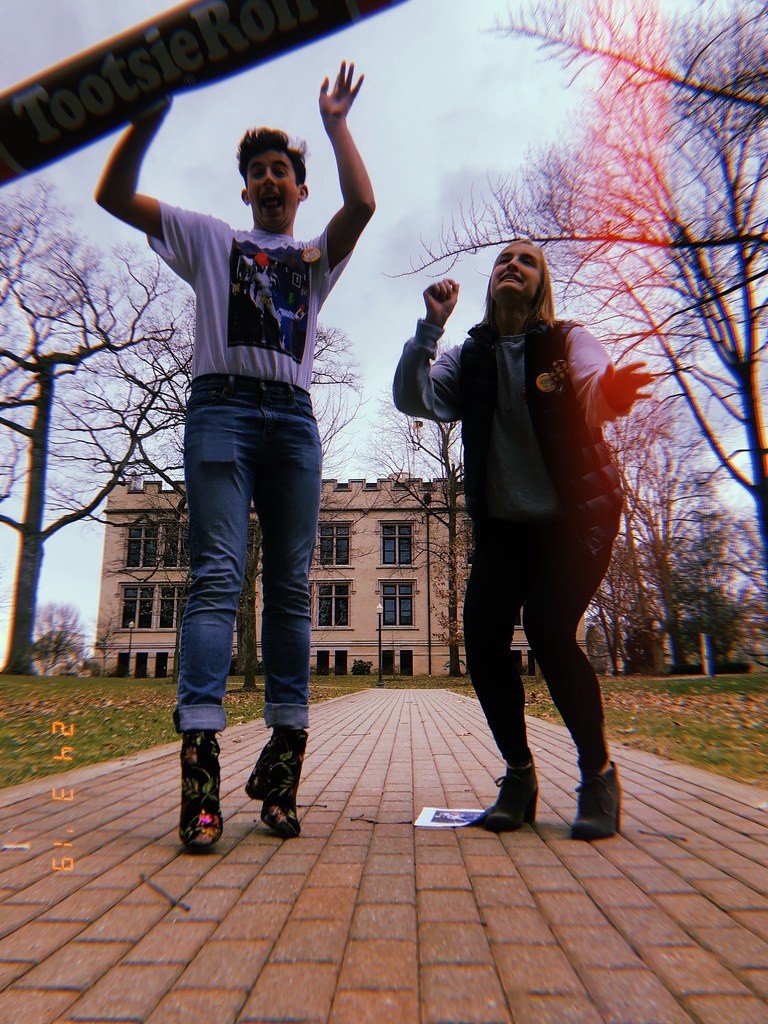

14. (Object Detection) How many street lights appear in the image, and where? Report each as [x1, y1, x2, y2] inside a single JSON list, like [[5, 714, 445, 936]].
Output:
[[124, 621, 135, 678], [376, 603, 387, 687]]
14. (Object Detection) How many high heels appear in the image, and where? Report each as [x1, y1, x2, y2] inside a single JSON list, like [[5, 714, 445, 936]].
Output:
[[246, 728, 309, 839], [569, 760, 621, 840], [179, 730, 223, 852], [485, 763, 539, 832]]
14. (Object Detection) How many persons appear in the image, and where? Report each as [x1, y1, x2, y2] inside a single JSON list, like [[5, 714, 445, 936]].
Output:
[[393, 240, 653, 840], [96, 62, 376, 848]]
